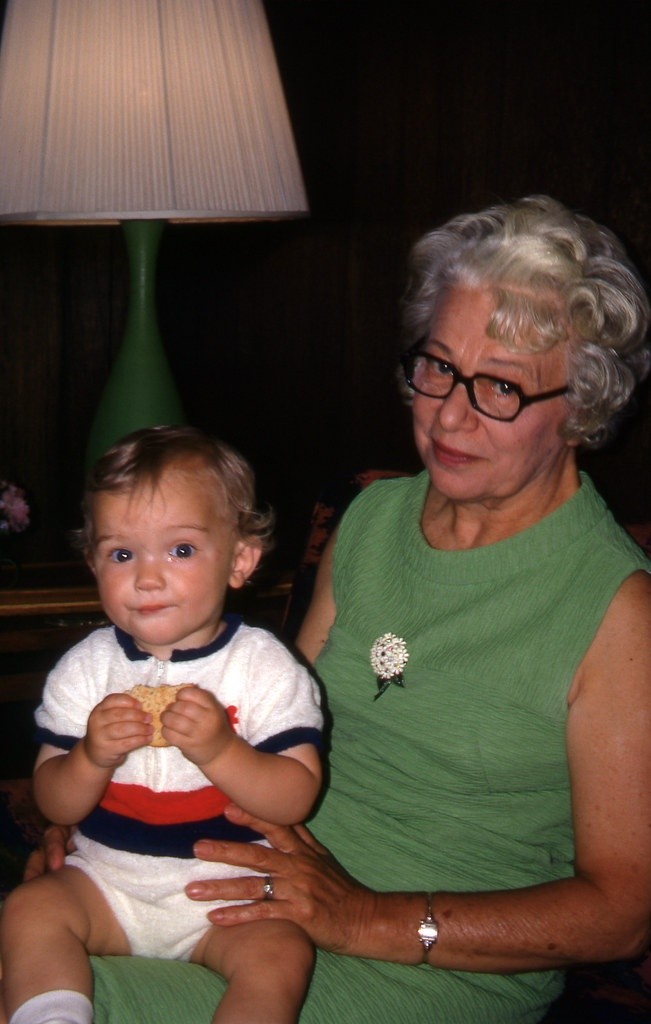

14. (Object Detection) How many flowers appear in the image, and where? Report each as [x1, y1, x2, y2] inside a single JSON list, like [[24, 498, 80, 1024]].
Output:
[[0, 478, 32, 537]]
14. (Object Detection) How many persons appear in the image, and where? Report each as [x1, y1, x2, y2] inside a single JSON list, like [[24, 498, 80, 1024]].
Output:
[[25, 196, 650, 1023], [0, 426, 327, 1023]]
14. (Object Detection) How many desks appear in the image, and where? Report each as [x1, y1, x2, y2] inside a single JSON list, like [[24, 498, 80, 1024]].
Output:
[[0, 528, 295, 703]]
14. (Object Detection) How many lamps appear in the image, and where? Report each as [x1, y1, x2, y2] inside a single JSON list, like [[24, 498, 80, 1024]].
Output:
[[0, 0, 312, 485]]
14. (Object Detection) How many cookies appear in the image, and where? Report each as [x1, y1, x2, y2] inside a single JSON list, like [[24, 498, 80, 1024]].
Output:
[[123, 682, 197, 746]]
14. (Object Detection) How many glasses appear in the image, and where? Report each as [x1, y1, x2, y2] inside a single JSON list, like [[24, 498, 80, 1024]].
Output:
[[400, 336, 570, 421]]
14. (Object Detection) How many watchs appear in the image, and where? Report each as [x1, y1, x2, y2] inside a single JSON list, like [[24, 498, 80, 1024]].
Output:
[[418, 892, 438, 963]]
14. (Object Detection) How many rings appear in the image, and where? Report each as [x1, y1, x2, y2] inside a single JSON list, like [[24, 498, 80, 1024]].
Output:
[[264, 877, 273, 901]]
